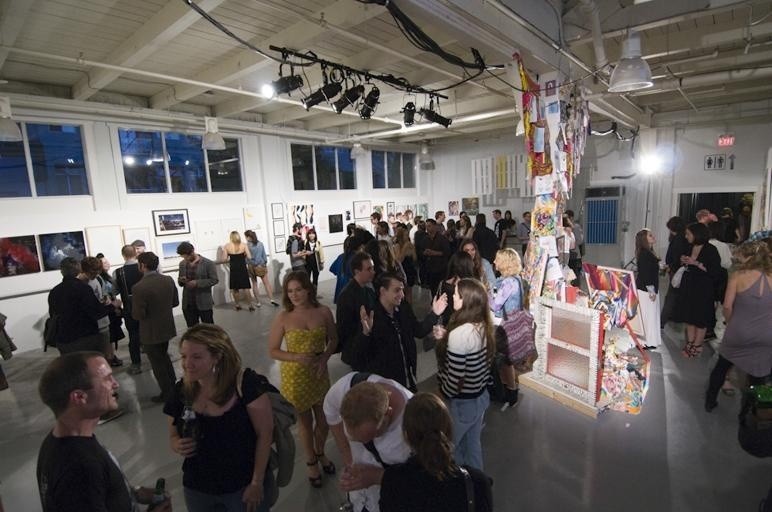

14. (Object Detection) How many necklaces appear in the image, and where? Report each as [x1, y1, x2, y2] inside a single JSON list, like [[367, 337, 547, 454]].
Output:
[[195, 395, 211, 408]]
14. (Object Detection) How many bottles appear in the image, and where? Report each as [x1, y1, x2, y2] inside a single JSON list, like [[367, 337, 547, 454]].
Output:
[[148, 478, 167, 512], [180, 401, 199, 443]]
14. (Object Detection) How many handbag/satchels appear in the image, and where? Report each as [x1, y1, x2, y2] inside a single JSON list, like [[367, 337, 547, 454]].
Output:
[[43, 313, 63, 348], [502, 308, 537, 365]]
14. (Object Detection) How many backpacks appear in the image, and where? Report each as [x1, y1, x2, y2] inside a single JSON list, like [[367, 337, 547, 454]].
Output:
[[236, 368, 298, 489]]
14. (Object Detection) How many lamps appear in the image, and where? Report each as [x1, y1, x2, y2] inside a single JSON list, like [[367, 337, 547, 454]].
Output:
[[208, 156, 239, 176], [153, 139, 172, 162], [252, 40, 456, 133], [417, 141, 437, 172], [348, 134, 367, 162], [1, 88, 24, 144], [197, 115, 228, 151], [597, 26, 657, 95]]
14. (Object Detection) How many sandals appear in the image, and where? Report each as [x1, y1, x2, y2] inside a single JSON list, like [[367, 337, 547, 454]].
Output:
[[315, 452, 336, 475], [683, 340, 703, 359], [306, 459, 323, 489]]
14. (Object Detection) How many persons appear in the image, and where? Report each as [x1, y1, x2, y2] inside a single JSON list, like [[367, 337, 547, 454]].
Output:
[[35, 351, 175, 512], [1, 314, 17, 397], [318, 367, 416, 511], [44, 199, 771, 478], [163, 325, 293, 510], [379, 394, 492, 510]]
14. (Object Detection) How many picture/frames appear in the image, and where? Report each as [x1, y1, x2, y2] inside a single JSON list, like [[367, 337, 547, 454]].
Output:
[[150, 208, 192, 238], [270, 202, 289, 255], [386, 201, 394, 218], [351, 199, 373, 234]]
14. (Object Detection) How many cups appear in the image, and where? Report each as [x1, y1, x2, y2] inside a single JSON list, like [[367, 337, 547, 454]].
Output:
[[566, 286, 580, 306], [433, 316, 441, 339]]
